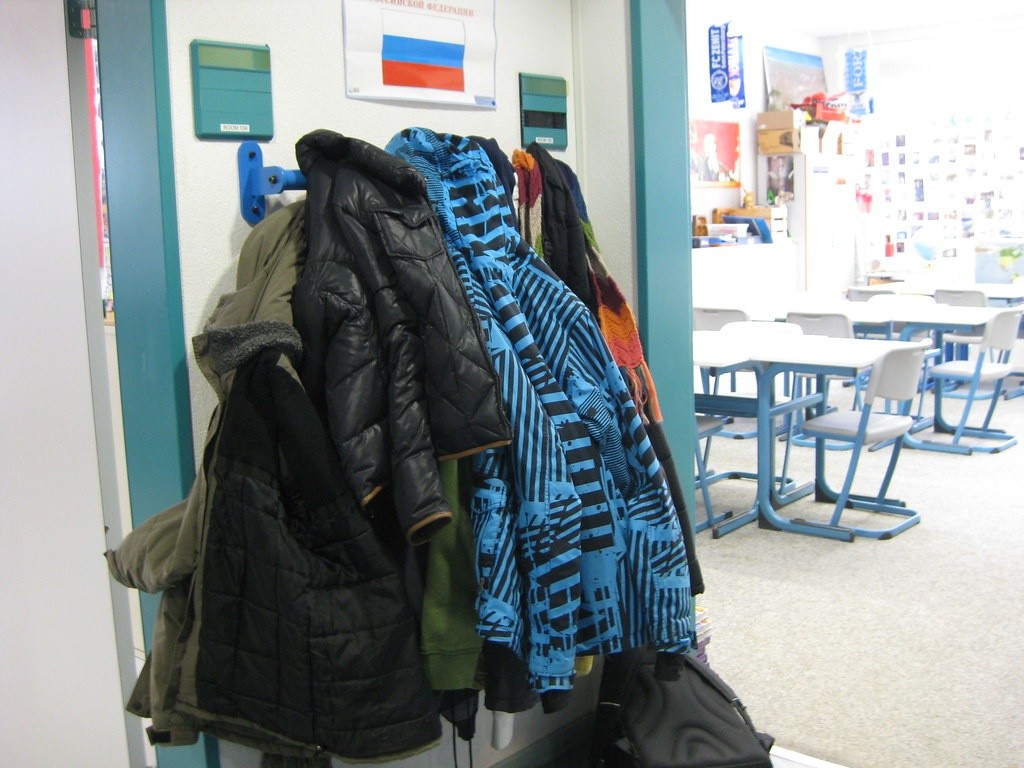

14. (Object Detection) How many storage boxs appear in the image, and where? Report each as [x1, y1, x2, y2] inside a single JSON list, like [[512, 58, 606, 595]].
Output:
[[706, 224, 749, 238], [730, 208, 788, 242], [757, 110, 806, 152]]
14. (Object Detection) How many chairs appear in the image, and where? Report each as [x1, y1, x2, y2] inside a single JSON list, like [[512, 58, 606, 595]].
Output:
[[692, 290, 1024, 542]]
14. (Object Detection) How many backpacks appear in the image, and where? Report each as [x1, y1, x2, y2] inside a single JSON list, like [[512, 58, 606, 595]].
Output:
[[588, 641, 774, 768]]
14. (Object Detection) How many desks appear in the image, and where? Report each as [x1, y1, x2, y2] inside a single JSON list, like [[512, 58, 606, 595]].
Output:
[[857, 283, 1024, 399], [693, 331, 921, 539], [775, 301, 1017, 452]]
